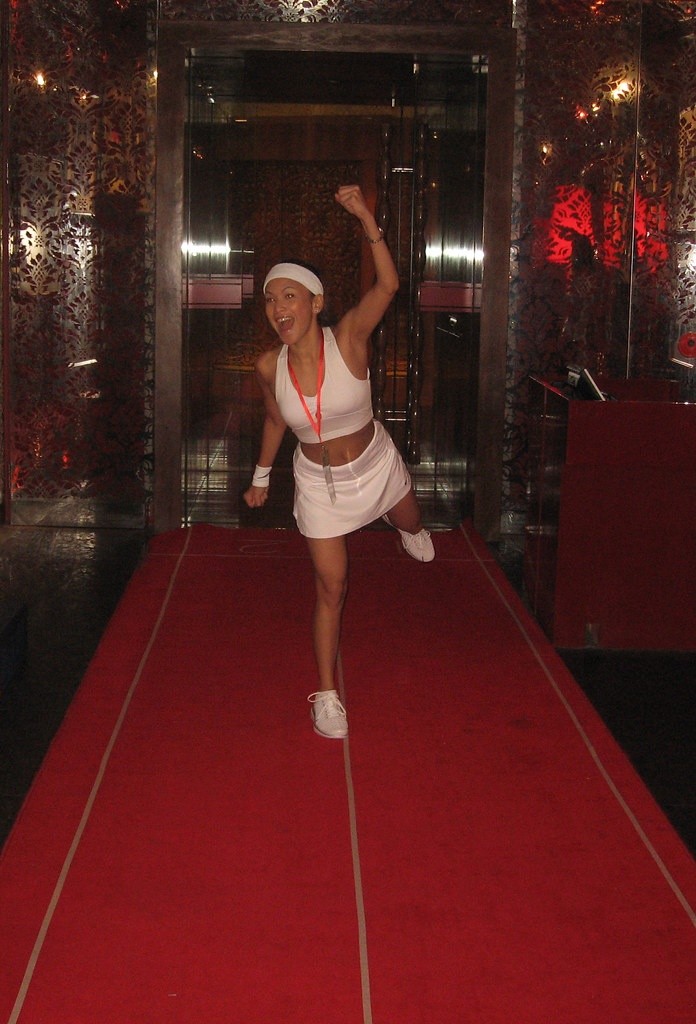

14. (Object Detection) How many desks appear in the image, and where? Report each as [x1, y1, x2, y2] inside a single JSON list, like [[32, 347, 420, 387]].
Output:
[[526, 372, 696, 651]]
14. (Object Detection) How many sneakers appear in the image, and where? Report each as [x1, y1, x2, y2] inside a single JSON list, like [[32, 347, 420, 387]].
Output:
[[383, 514, 435, 563], [307, 691, 349, 739]]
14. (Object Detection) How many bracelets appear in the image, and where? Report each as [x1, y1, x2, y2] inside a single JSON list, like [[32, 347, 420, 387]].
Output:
[[251, 462, 272, 487]]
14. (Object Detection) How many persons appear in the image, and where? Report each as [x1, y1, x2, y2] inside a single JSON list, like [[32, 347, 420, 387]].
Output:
[[244, 185, 433, 739]]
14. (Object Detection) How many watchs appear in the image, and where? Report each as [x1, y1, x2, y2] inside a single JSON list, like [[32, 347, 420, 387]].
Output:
[[366, 227, 384, 243]]
